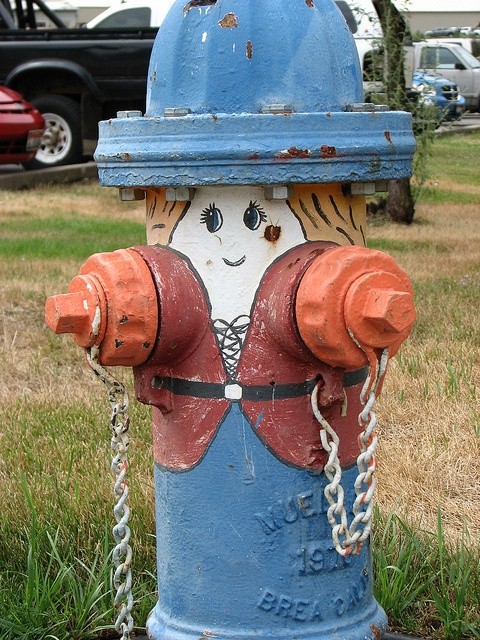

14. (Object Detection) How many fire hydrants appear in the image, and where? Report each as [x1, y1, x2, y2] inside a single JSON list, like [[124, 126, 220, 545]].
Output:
[[42, 1, 418, 640]]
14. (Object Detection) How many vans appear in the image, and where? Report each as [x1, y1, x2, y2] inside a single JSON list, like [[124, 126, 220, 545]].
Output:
[[79, 0, 391, 103]]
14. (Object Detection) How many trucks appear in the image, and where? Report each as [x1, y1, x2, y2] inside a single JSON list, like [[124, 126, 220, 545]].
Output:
[[361, 41, 480, 121]]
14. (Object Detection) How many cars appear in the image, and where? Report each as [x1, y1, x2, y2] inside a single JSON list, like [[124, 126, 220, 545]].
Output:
[[0, 83, 46, 169], [411, 72, 467, 130], [423, 26, 455, 37]]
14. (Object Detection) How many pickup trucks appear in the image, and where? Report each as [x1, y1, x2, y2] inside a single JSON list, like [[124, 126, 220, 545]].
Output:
[[0, 0, 160, 171]]
